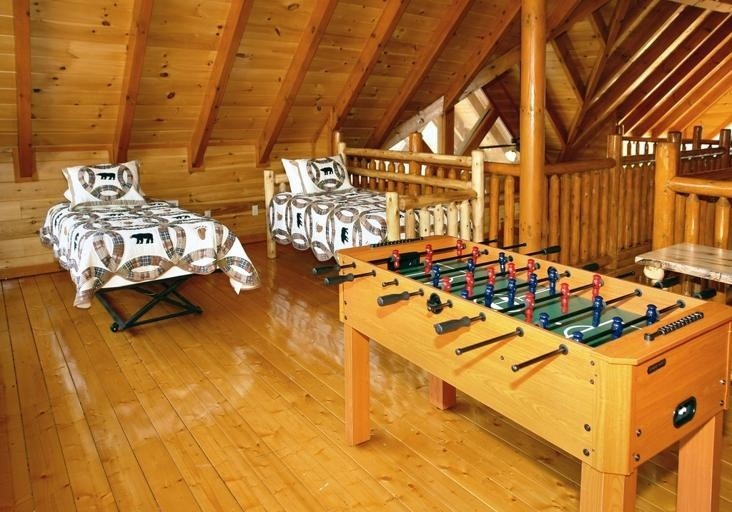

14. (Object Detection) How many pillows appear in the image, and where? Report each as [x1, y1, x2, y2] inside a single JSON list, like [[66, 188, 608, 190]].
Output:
[[281, 153, 358, 199], [61, 158, 150, 211]]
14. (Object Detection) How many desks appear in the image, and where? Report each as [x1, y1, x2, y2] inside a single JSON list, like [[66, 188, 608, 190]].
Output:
[[635, 240, 732, 294]]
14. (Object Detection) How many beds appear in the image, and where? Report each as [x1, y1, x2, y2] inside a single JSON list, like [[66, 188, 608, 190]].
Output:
[[263, 150, 496, 262], [37, 201, 262, 334]]
